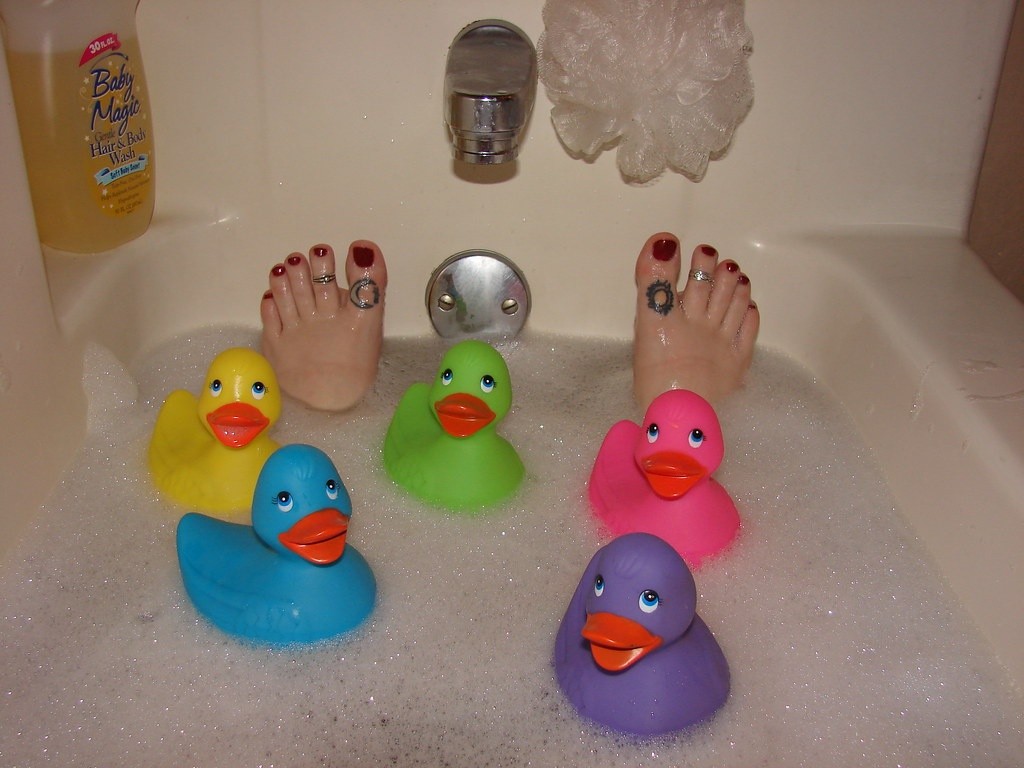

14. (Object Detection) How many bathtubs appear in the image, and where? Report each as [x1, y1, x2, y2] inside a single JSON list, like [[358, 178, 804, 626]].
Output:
[[0, 198, 1024, 763]]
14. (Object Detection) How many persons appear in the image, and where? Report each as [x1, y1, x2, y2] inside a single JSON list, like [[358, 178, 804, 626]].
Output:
[[259, 233, 760, 413]]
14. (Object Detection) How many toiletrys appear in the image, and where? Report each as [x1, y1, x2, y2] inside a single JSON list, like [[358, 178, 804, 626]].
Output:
[[0, 0, 156, 252]]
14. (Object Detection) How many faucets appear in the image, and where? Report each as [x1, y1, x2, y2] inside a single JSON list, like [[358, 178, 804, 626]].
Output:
[[441, 17, 539, 169]]
